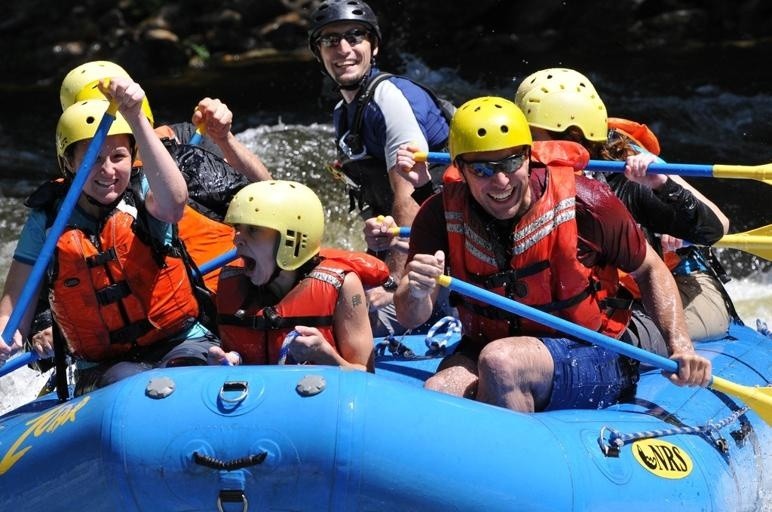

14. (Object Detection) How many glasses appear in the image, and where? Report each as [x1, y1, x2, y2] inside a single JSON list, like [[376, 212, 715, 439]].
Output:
[[462, 150, 532, 179], [314, 26, 372, 49]]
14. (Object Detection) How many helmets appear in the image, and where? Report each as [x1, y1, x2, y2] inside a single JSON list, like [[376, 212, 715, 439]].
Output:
[[223, 179, 325, 273], [309, 0, 385, 43], [514, 67, 609, 146], [447, 91, 535, 164], [55, 58, 154, 170]]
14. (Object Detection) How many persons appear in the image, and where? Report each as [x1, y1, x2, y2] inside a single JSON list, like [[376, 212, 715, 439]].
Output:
[[521, 77, 725, 311], [204, 178, 375, 370], [307, 0, 449, 336], [0, 78, 222, 398], [58, 61, 273, 222], [393, 94, 713, 413], [512, 68, 732, 374]]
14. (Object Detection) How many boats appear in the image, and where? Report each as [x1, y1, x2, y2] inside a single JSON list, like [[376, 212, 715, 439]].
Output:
[[0, 318, 771, 512]]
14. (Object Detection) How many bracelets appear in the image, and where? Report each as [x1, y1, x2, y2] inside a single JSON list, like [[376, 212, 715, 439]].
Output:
[[382, 279, 397, 293]]
